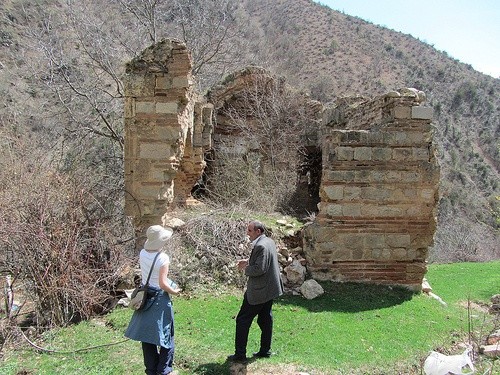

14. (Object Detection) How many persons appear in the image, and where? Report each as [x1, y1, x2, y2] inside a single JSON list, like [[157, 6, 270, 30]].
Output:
[[123, 224, 184, 375], [227, 221, 284, 361]]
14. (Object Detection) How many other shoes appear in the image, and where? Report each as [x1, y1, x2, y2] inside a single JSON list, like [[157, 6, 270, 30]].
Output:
[[167, 370, 178, 375], [253, 352, 271, 357], [229, 354, 247, 360]]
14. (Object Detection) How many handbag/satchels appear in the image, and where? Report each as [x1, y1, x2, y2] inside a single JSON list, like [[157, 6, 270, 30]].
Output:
[[131, 285, 147, 310]]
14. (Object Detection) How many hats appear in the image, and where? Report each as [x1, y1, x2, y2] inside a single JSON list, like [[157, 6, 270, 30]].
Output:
[[143, 225, 173, 250]]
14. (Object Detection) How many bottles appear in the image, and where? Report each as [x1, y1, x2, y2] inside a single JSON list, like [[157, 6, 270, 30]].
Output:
[[166, 277, 178, 290]]
